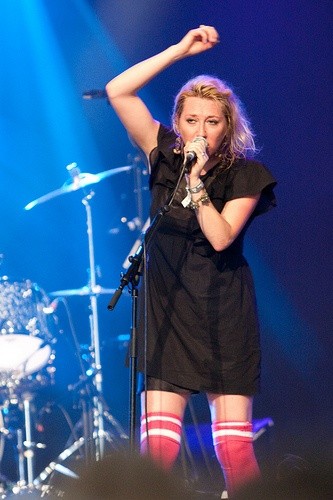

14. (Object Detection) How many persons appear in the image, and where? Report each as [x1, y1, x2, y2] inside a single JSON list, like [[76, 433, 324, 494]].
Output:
[[105, 24, 277, 500]]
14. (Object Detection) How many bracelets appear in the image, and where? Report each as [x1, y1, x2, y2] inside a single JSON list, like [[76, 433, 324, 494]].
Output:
[[179, 181, 209, 209]]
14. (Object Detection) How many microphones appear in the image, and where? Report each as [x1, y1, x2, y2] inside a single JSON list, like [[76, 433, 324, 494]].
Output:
[[71, 363, 98, 391], [50, 297, 61, 311], [186, 137, 207, 162]]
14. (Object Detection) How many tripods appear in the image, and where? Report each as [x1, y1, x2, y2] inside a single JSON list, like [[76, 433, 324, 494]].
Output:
[[32, 195, 131, 489]]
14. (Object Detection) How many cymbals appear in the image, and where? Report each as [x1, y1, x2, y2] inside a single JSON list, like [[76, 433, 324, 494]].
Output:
[[48, 283, 124, 296], [24, 162, 132, 211]]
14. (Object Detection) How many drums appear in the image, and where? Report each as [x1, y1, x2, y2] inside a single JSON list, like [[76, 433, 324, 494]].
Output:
[[0, 333, 57, 403]]
